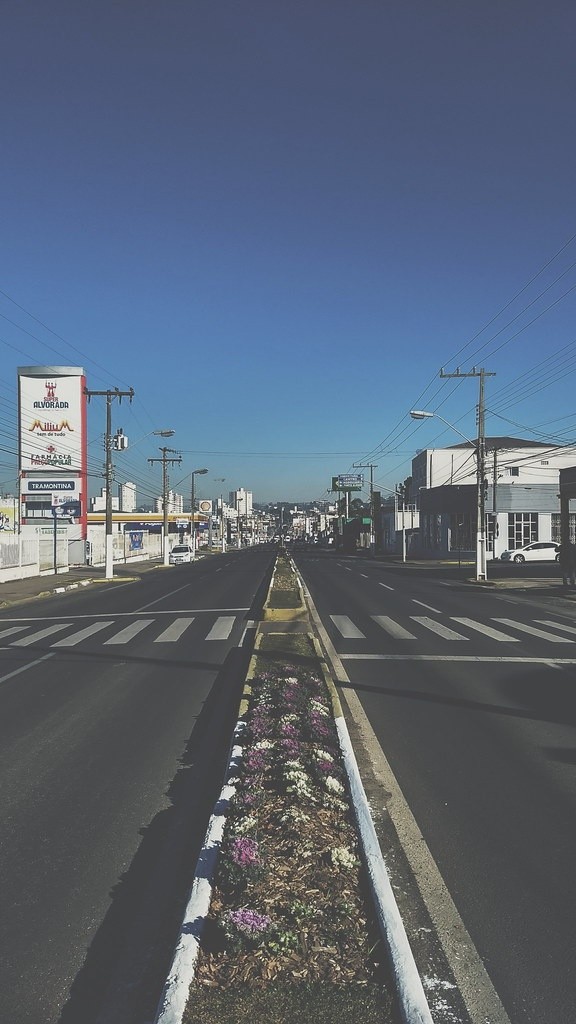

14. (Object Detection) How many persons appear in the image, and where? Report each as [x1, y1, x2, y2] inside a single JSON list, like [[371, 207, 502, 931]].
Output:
[[555, 538, 576, 586]]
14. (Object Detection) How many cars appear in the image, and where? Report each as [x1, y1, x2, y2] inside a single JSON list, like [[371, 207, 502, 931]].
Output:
[[270, 532, 284, 544], [314, 539, 318, 544], [169, 544, 195, 566], [498, 541, 561, 564], [285, 536, 290, 542]]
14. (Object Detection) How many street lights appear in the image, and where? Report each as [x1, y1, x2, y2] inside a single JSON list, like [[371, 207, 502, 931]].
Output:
[[163, 469, 209, 566], [105, 429, 175, 578], [221, 498, 243, 552], [410, 411, 482, 580]]
